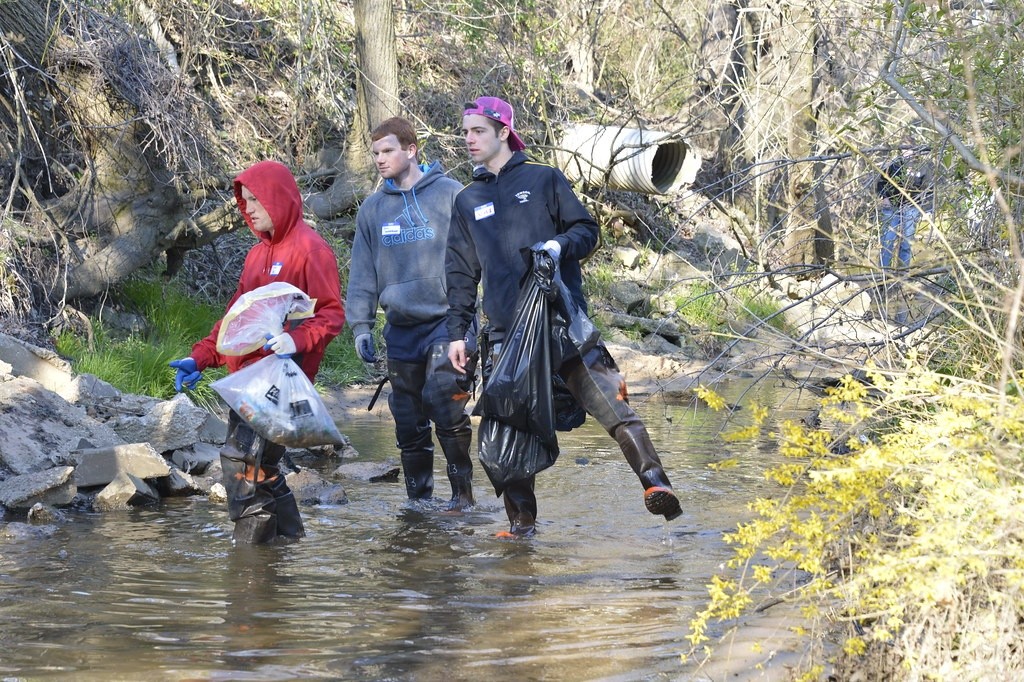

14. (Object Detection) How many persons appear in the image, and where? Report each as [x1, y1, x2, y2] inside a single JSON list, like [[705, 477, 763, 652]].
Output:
[[344, 115, 487, 512], [445, 95, 684, 539], [168, 159, 345, 548], [871, 134, 934, 299]]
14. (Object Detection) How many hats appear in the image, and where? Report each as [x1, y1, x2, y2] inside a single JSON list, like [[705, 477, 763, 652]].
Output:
[[464, 96, 527, 151]]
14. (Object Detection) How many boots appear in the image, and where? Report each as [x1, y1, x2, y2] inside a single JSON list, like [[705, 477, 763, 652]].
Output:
[[400, 451, 434, 501], [619, 430, 684, 521], [440, 434, 477, 517], [498, 473, 541, 539]]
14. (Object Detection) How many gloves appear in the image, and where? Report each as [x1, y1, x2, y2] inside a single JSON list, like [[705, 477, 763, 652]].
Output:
[[263, 332, 296, 359], [356, 333, 377, 363], [169, 357, 202, 393], [538, 239, 561, 268]]
